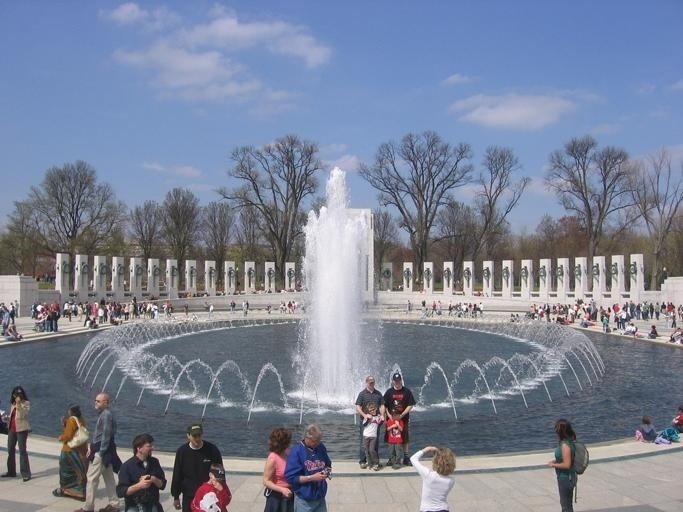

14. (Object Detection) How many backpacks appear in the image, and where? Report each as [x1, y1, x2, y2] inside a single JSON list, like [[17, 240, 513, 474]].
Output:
[[662, 428, 680, 440], [571, 441, 588, 473]]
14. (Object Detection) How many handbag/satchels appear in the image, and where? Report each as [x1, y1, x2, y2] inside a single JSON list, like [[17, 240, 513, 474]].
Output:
[[67, 425, 89, 449]]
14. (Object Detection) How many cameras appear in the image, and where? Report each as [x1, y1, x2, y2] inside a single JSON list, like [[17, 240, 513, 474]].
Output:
[[320, 466, 332, 480]]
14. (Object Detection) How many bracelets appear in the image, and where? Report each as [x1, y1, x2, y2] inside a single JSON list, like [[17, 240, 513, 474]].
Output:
[[307, 475, 311, 482]]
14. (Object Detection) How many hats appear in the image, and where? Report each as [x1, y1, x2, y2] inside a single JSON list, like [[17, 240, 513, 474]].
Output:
[[367, 376, 375, 384], [187, 425, 202, 436], [210, 469, 226, 482], [394, 375, 402, 381]]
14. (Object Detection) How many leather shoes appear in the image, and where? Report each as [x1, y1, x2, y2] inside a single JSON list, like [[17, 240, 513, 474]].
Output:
[[98, 505, 120, 512]]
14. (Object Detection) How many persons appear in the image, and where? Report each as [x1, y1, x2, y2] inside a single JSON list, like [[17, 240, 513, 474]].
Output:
[[546, 417, 578, 511], [410, 444, 457, 512], [267, 305, 271, 314], [258, 424, 294, 510], [283, 423, 332, 512], [612, 300, 683, 344], [116, 434, 166, 511], [382, 408, 407, 471], [525, 299, 598, 328], [407, 300, 411, 311], [243, 300, 249, 316], [231, 300, 236, 312], [383, 370, 416, 467], [50, 403, 91, 502], [0, 300, 21, 341], [163, 303, 172, 320], [0, 385, 33, 482], [599, 306, 611, 333], [170, 422, 225, 511], [204, 302, 214, 315], [421, 299, 441, 318], [510, 314, 519, 322], [359, 400, 384, 471], [184, 304, 189, 315], [31, 300, 60, 332], [74, 390, 123, 511], [280, 300, 304, 314], [640, 413, 659, 444], [353, 374, 386, 469], [449, 302, 483, 318], [190, 461, 231, 512], [670, 405, 682, 433], [63, 298, 159, 329]]
